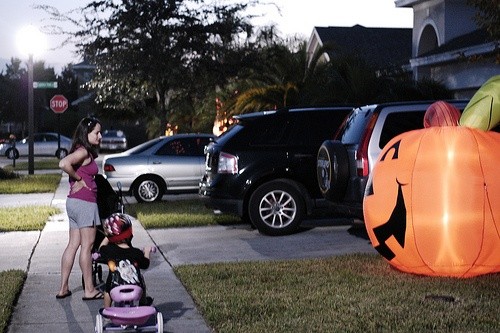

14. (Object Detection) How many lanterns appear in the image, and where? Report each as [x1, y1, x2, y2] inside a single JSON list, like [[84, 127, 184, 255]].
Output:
[[361, 76, 500, 279]]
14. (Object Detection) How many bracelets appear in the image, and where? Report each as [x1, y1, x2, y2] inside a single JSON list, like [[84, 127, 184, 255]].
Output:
[[77, 176, 82, 182]]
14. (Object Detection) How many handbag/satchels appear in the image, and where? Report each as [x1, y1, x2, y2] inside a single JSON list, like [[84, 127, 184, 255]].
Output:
[[94, 174, 119, 219]]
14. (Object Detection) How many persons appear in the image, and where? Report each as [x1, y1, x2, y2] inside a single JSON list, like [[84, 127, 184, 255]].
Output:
[[94, 213, 153, 320], [56, 117, 106, 301]]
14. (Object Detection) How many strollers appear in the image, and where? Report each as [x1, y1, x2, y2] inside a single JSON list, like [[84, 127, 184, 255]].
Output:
[[81, 174, 127, 290]]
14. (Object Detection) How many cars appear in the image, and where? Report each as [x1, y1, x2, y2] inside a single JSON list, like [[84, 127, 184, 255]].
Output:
[[101, 132, 220, 202], [0, 131, 75, 161]]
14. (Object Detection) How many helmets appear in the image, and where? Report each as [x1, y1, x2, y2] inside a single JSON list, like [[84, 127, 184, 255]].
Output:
[[103, 212, 133, 243]]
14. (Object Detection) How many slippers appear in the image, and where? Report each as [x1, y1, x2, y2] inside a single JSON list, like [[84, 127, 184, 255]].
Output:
[[56, 289, 72, 298], [82, 291, 104, 300]]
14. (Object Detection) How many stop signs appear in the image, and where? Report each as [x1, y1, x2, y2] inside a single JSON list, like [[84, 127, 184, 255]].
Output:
[[49, 95, 69, 113]]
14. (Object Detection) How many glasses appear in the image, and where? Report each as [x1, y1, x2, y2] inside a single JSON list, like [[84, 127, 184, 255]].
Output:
[[88, 116, 96, 125]]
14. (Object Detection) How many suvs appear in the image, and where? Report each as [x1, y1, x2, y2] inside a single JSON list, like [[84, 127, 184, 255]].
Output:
[[197, 106, 358, 237], [316, 98, 474, 222], [99, 129, 128, 152]]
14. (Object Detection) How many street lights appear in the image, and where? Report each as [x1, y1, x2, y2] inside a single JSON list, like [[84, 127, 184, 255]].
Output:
[[18, 25, 51, 174]]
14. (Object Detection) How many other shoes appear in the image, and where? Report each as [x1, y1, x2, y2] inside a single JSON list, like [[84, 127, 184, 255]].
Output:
[[98, 308, 112, 322], [138, 296, 153, 306]]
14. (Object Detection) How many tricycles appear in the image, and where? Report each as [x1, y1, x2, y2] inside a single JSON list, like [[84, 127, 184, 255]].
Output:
[[92, 245, 165, 333]]
